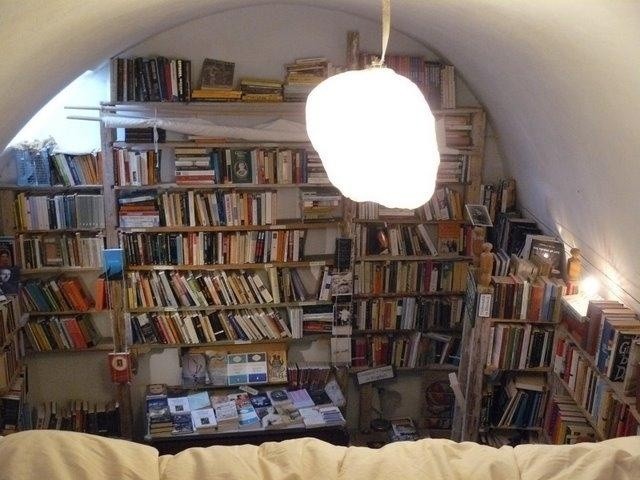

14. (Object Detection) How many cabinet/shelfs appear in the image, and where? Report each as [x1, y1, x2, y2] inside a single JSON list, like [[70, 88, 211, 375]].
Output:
[[474, 276, 640, 446], [0, 103, 490, 433]]
